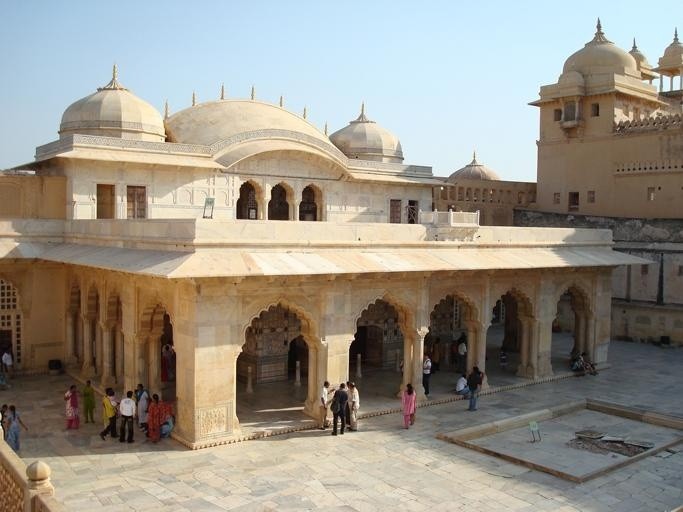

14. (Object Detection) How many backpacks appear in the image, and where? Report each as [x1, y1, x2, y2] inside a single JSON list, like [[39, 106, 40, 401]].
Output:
[[430, 362, 436, 374], [330, 390, 341, 413]]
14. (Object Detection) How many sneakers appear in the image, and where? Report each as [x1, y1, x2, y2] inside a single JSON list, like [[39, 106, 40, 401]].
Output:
[[111, 433, 119, 438], [99, 431, 107, 440], [119, 438, 136, 443], [340, 430, 345, 434], [318, 423, 329, 430], [346, 426, 357, 432], [331, 431, 338, 436]]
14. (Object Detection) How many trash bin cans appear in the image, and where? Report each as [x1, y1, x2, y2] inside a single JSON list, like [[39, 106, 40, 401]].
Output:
[[48, 359, 61, 376]]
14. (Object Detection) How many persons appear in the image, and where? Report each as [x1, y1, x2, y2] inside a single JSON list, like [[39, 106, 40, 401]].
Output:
[[570, 352, 600, 376], [161, 339, 174, 355], [455, 373, 472, 399], [422, 353, 432, 395], [97, 383, 178, 445], [0, 404, 7, 440], [498, 349, 508, 369], [62, 384, 81, 431], [426, 332, 467, 373], [401, 383, 416, 430], [79, 379, 96, 424], [2, 348, 14, 389], [314, 379, 360, 436], [160, 344, 175, 382], [465, 365, 483, 413], [6, 404, 29, 452]]
